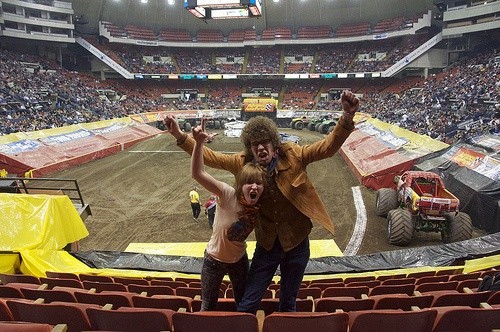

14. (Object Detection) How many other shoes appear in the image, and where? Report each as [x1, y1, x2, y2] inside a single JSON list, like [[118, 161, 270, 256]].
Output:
[[193, 217, 199, 223]]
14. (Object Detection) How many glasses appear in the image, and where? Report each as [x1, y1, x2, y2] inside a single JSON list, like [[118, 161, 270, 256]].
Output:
[[249, 138, 272, 148]]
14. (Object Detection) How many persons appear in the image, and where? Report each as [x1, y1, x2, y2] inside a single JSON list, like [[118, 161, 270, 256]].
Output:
[[162, 89, 359, 314], [190, 125, 267, 312], [0, 32, 500, 228]]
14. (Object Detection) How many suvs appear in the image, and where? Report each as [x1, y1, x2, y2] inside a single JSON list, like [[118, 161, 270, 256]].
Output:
[[158, 114, 230, 133], [375, 171, 473, 246], [292, 114, 337, 135]]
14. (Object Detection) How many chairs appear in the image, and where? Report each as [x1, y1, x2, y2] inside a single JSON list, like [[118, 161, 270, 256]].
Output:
[[46, 17, 458, 112], [0, 266, 500, 332]]
